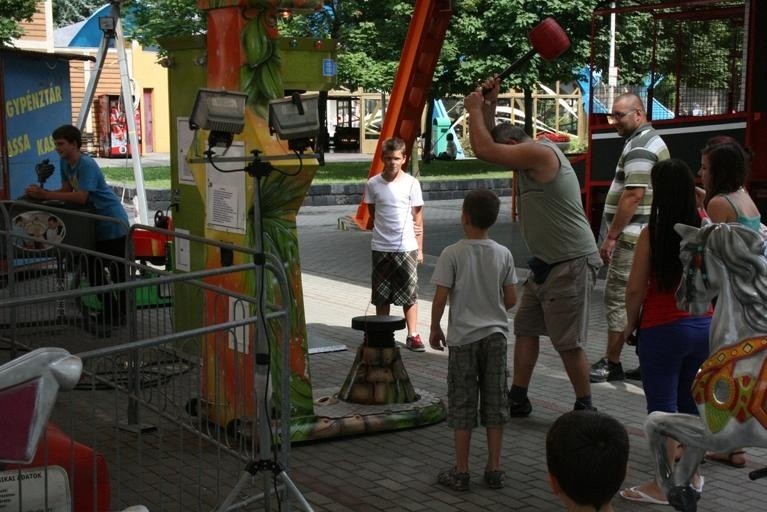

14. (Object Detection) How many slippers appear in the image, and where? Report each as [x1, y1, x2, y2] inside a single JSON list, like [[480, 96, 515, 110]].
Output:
[[619, 486, 670, 506], [688, 476, 705, 494]]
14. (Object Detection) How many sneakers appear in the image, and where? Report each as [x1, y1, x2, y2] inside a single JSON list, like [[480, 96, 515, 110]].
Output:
[[438, 466, 471, 493], [405, 334, 427, 353], [483, 464, 506, 490], [508, 396, 532, 419], [571, 400, 600, 412]]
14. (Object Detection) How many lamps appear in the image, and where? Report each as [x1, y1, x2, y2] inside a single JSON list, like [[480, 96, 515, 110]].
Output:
[[187, 87, 248, 149], [268, 92, 320, 152], [97, 2, 121, 39]]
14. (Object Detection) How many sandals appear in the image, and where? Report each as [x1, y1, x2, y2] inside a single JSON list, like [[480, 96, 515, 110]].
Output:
[[704, 449, 747, 469]]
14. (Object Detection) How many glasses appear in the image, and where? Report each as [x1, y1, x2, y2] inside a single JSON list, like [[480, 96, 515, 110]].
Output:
[[611, 109, 636, 118]]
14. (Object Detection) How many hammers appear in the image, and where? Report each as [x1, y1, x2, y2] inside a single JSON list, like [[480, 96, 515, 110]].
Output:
[[482, 17, 572, 95]]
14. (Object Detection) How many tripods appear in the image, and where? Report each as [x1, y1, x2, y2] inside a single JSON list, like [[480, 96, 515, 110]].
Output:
[[215, 162, 316, 512]]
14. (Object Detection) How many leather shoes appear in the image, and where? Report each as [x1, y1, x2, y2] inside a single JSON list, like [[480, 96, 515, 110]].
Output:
[[625, 366, 642, 381], [589, 357, 626, 384]]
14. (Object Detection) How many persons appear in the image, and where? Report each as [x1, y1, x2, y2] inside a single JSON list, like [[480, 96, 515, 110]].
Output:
[[590, 92, 670, 384], [430, 191, 517, 490], [463, 75, 603, 417], [695, 140, 761, 467], [541, 410, 631, 510], [678, 100, 718, 117], [27, 124, 132, 330], [620, 160, 705, 504], [434, 132, 457, 161], [364, 136, 424, 352]]
